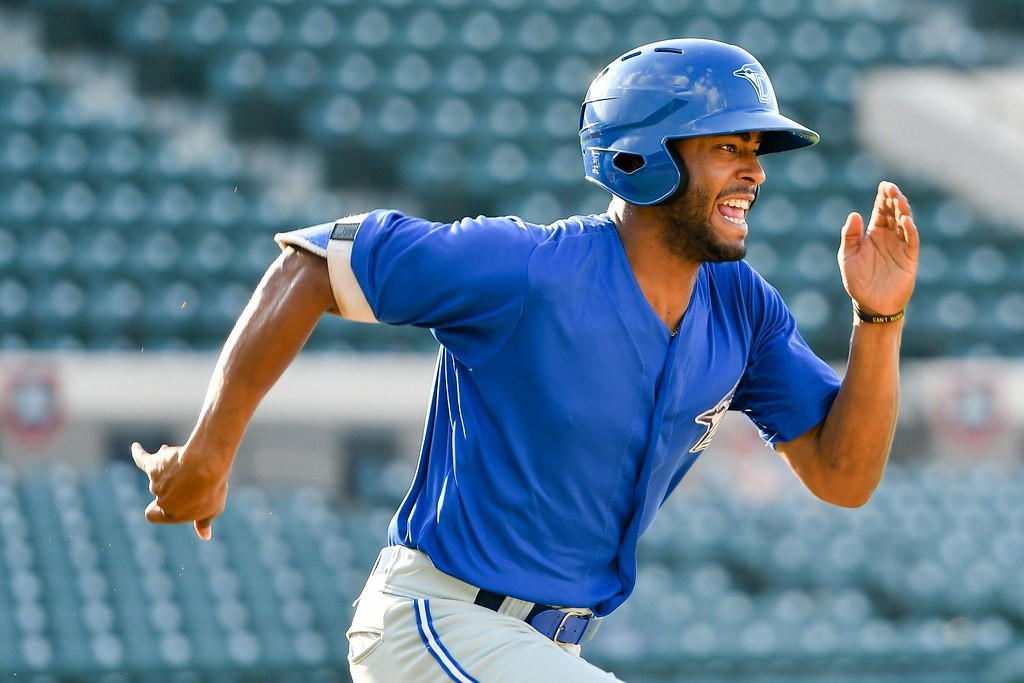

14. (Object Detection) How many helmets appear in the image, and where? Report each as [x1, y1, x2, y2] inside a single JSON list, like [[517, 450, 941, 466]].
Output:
[[578, 38, 819, 205]]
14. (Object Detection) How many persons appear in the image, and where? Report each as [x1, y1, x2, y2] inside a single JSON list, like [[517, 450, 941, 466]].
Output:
[[131, 38, 920, 683]]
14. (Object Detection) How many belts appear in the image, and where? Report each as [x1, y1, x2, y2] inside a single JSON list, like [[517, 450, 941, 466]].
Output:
[[474, 589, 602, 647]]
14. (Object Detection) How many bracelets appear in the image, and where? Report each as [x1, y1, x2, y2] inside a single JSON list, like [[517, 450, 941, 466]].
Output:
[[853, 303, 904, 324]]
